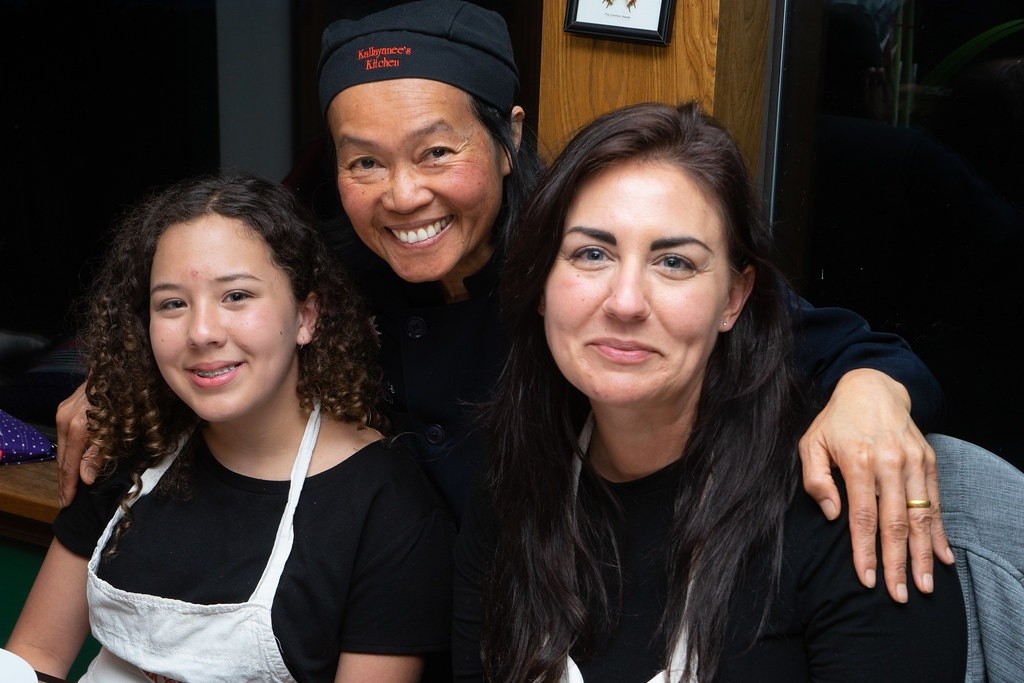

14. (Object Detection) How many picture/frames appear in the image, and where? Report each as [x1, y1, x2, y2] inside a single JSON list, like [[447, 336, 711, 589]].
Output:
[[563, 0, 677, 47]]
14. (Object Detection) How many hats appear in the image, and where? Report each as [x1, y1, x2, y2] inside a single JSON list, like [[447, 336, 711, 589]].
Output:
[[318, 0, 519, 116]]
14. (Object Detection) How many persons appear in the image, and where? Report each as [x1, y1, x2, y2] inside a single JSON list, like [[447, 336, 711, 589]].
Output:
[[3, 171, 458, 683], [54, 0, 957, 606], [451, 99, 968, 683]]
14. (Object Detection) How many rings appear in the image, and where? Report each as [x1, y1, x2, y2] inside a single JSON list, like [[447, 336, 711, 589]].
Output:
[[906, 499, 931, 508]]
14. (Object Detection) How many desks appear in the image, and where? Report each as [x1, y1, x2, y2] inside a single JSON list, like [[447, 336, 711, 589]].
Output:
[[0, 422, 60, 548]]
